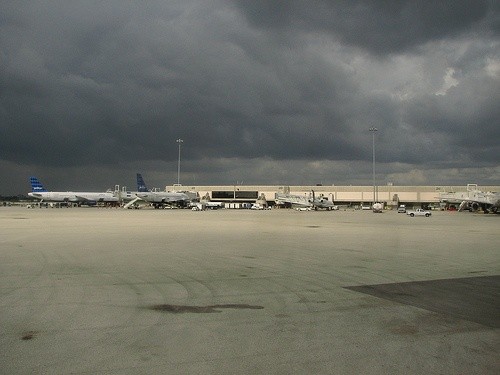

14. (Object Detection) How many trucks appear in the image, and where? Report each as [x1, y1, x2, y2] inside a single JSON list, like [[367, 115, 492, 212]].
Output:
[[397, 204, 406, 213]]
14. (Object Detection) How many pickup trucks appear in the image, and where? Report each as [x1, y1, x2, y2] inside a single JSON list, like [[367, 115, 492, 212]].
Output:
[[407, 208, 431, 217]]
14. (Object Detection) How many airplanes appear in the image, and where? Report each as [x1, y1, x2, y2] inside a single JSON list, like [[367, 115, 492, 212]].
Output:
[[117, 174, 191, 208], [309, 189, 335, 211], [27, 175, 125, 202]]
